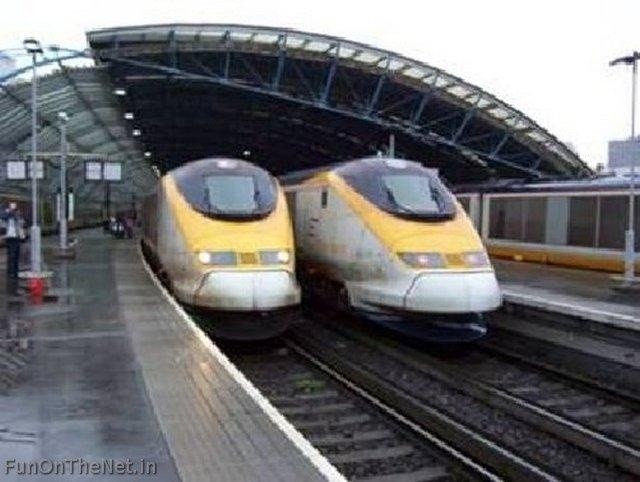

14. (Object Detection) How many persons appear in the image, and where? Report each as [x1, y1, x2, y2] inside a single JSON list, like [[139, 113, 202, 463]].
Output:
[[0, 202, 30, 279], [98, 212, 142, 240]]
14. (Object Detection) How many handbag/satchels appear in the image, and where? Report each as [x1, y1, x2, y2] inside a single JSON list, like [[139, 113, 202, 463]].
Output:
[[18, 229, 28, 239]]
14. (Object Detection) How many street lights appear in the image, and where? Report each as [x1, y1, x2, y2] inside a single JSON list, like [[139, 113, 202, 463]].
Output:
[[23, 38, 69, 272], [610, 51, 639, 275]]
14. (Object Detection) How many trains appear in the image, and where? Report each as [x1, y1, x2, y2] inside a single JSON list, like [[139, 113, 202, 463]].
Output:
[[445, 178, 639, 276], [276, 156, 502, 360], [144, 158, 302, 342]]
